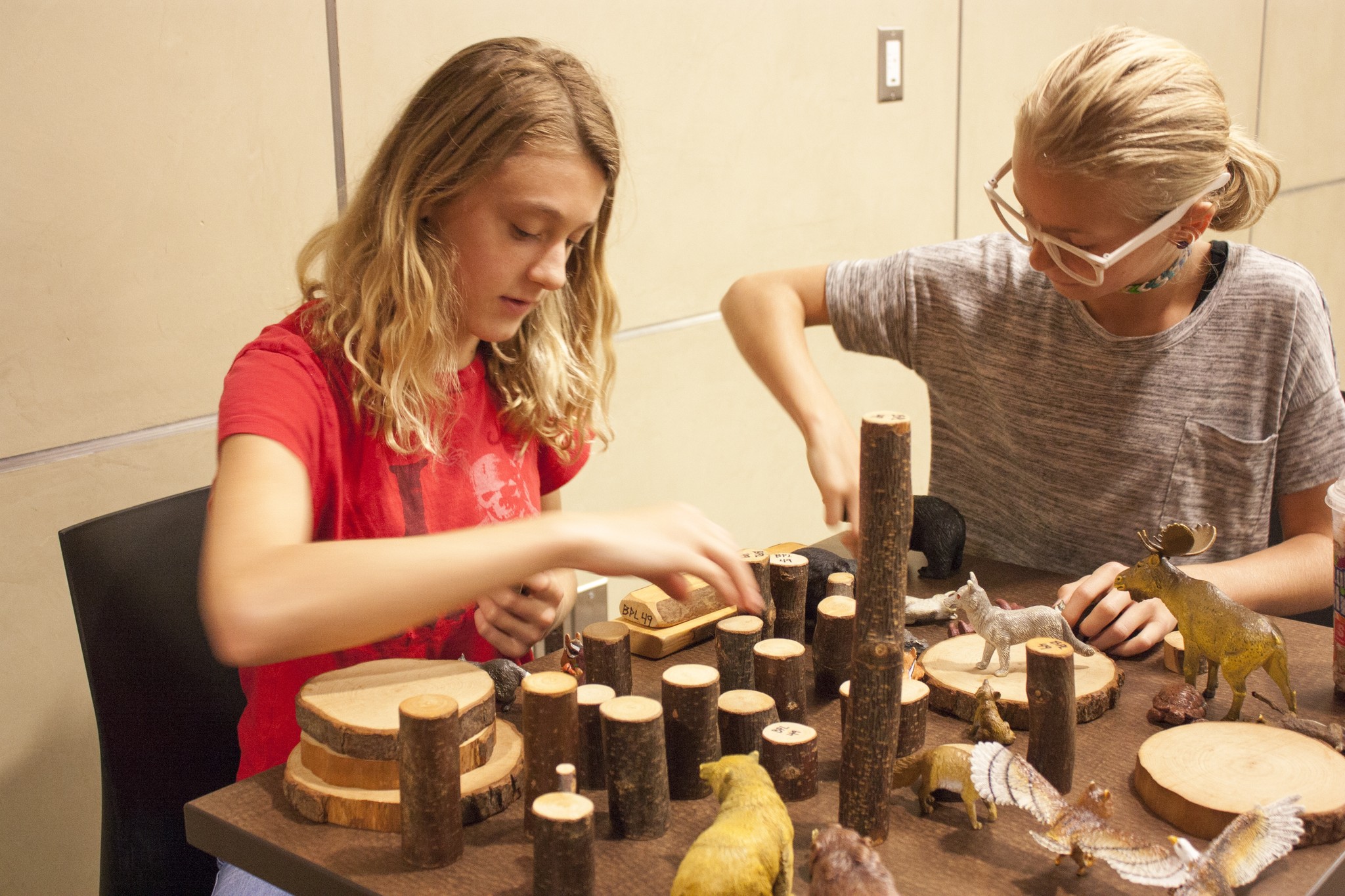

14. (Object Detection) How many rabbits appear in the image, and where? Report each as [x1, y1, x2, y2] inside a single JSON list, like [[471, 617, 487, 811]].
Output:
[[456, 653, 531, 712]]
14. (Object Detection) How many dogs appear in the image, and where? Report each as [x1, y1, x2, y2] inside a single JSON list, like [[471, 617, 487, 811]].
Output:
[[942, 571, 1094, 678], [810, 823, 899, 896]]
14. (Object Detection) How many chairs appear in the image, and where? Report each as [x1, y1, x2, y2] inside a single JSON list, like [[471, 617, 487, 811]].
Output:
[[56, 484, 270, 895]]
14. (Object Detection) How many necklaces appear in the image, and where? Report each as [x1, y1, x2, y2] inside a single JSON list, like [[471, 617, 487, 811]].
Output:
[[1117, 244, 1192, 294]]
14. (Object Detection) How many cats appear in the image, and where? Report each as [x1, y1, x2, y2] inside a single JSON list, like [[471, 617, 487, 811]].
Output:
[[892, 742, 997, 829]]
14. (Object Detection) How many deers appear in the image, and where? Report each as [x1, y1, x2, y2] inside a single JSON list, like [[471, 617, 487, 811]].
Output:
[[1113, 522, 1298, 723]]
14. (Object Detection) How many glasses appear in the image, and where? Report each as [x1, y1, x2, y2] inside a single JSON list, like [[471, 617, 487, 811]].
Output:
[[983, 150, 1232, 286]]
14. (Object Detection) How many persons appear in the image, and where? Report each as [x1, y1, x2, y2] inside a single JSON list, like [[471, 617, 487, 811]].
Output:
[[192, 36, 769, 896], [723, 30, 1344, 659]]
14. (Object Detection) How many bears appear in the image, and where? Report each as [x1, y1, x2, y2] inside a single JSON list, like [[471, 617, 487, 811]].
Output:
[[909, 493, 966, 578], [962, 680, 1015, 746], [667, 749, 794, 896]]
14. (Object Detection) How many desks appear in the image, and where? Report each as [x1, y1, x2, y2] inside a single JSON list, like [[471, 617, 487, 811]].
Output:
[[182, 532, 1344, 895]]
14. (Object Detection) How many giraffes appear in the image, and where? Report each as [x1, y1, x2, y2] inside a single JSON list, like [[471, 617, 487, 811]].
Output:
[[790, 546, 959, 639]]
